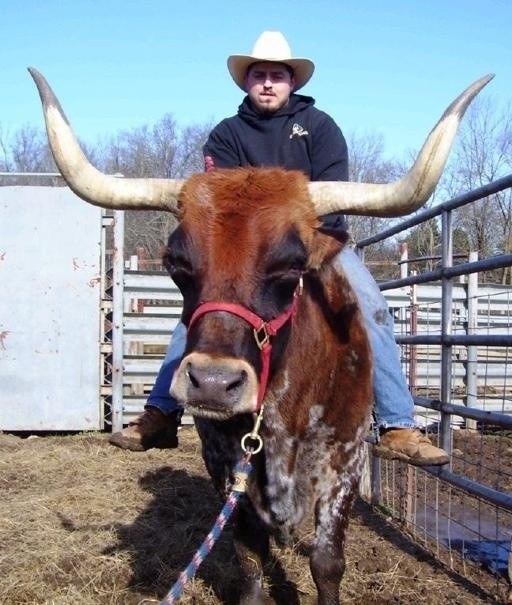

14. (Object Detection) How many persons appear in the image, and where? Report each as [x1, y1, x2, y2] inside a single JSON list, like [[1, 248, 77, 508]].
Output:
[[109, 29, 450, 464]]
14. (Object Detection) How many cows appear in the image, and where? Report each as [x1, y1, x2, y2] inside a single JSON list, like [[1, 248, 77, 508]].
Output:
[[27, 66, 497, 605]]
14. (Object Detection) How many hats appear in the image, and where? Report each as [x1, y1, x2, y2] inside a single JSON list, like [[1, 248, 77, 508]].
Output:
[[226, 30, 315, 92]]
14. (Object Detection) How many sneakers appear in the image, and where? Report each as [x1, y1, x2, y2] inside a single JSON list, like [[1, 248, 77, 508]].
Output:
[[372, 427, 451, 466], [108, 406, 178, 451]]
